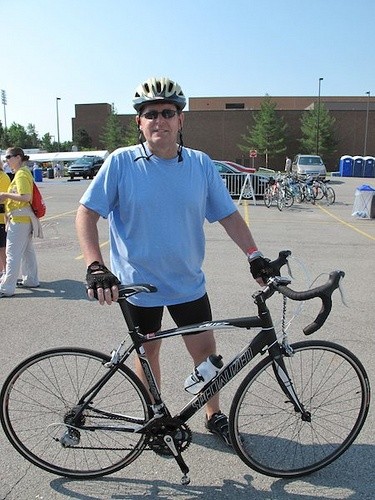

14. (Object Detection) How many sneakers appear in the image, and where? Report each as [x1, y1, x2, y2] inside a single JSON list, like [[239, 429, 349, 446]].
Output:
[[205, 410, 244, 446], [0, 292, 15, 298], [16, 281, 41, 288]]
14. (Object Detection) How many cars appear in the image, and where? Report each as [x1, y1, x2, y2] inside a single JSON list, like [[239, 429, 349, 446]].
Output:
[[212, 160, 270, 200]]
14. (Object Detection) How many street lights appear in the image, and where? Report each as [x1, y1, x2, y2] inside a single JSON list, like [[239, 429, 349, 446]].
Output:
[[56, 97, 61, 152], [363, 90, 371, 156], [1, 89, 7, 127], [316, 76, 324, 155]]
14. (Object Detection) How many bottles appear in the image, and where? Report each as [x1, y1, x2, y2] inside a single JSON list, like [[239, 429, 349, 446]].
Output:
[[184, 354, 224, 396]]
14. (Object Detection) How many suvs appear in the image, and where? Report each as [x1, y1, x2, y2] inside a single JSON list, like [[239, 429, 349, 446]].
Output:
[[68, 155, 104, 180]]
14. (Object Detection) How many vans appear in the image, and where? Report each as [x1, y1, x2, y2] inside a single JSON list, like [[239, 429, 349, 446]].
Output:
[[292, 154, 326, 182]]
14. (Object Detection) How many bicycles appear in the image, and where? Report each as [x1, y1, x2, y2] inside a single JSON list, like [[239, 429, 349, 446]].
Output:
[[263, 174, 336, 211], [0, 250, 371, 485]]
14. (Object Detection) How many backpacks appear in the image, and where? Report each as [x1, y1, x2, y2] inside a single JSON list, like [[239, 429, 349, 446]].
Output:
[[18, 169, 46, 218]]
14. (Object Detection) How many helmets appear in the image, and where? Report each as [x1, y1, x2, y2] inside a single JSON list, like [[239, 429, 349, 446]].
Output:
[[132, 76, 186, 112]]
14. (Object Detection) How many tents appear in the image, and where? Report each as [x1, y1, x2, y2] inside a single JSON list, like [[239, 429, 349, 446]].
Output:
[[0, 151, 109, 179]]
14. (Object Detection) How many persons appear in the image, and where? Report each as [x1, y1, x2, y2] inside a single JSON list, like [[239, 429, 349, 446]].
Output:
[[0, 146, 44, 300], [33, 163, 38, 169], [284, 157, 291, 172], [76, 76, 281, 446], [54, 161, 64, 179]]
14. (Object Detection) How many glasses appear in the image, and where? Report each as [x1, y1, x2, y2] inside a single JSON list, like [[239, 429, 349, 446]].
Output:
[[6, 154, 19, 159], [140, 110, 178, 119]]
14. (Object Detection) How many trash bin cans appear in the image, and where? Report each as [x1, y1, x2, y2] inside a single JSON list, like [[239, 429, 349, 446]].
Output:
[[47, 168, 54, 179], [340, 155, 375, 178], [33, 168, 42, 182]]
